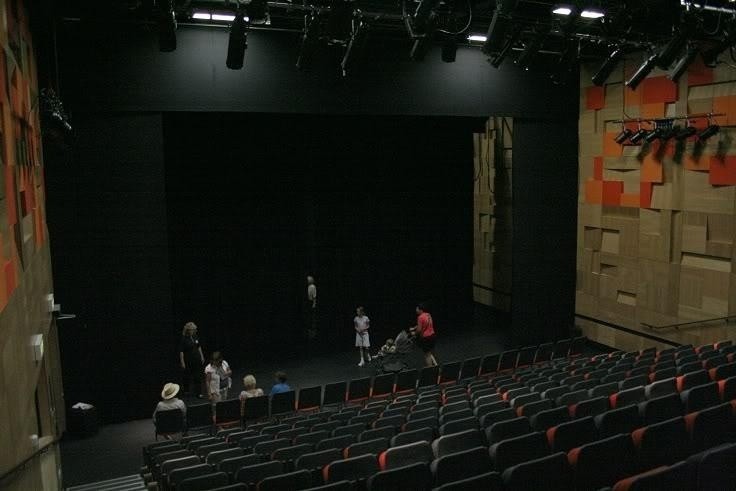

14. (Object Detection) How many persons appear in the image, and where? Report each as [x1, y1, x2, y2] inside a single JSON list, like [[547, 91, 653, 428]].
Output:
[[305, 276, 317, 338], [354, 306, 372, 367], [152, 383, 188, 440], [240, 374, 264, 417], [372, 303, 437, 367], [269, 371, 290, 402], [204, 351, 233, 436], [179, 322, 206, 399]]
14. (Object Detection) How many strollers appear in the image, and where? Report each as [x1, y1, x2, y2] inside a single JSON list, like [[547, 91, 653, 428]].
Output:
[[373, 329, 421, 373]]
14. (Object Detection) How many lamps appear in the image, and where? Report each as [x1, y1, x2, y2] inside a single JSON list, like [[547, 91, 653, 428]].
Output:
[[615, 115, 721, 145]]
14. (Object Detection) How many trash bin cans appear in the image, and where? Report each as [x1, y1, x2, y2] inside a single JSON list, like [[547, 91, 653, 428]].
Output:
[[70, 399, 98, 440]]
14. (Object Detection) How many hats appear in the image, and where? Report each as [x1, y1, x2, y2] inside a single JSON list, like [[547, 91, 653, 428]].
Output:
[[160, 382, 181, 400]]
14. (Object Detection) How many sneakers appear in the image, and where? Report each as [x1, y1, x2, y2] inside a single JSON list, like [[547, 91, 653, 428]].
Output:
[[357, 360, 366, 367], [366, 354, 372, 361]]
